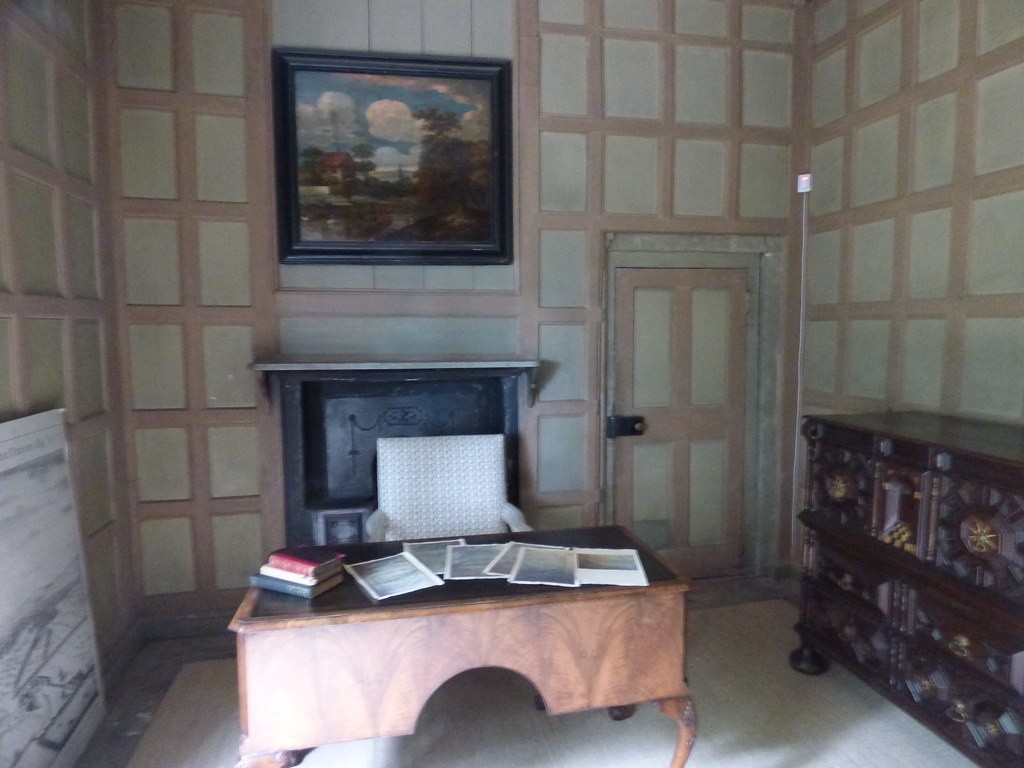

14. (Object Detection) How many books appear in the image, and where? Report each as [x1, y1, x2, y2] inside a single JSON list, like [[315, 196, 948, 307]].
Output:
[[250, 544, 345, 599]]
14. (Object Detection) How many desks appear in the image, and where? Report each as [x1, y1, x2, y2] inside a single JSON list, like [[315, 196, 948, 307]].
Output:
[[224, 523, 698, 768]]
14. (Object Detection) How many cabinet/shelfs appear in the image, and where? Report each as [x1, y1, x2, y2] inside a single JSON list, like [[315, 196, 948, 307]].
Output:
[[788, 409, 1024, 766]]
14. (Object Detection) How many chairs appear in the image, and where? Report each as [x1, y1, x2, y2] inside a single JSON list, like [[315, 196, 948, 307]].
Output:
[[363, 433, 533, 547]]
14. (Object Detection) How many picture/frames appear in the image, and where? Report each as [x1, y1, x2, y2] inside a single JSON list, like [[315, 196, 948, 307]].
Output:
[[271, 45, 514, 266]]
